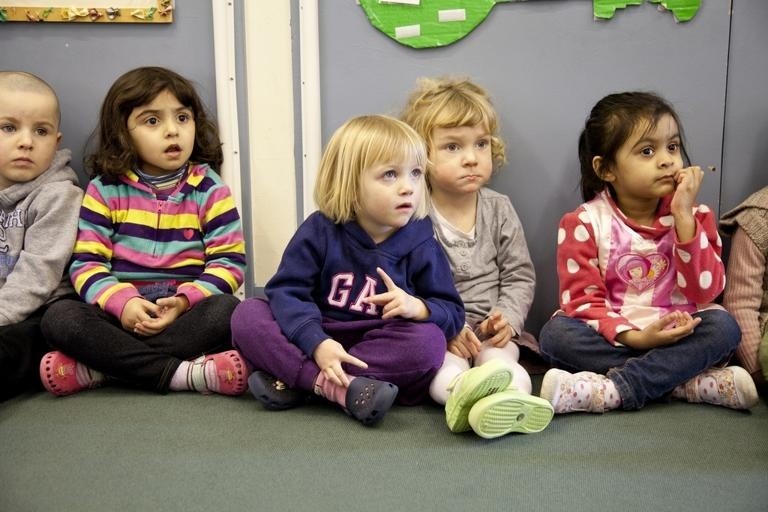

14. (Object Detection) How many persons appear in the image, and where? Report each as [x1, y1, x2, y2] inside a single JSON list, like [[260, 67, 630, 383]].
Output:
[[0, 66, 83, 403], [228, 112, 467, 429], [36, 66, 250, 398], [398, 70, 557, 443], [538, 88, 762, 417], [717, 183, 768, 397]]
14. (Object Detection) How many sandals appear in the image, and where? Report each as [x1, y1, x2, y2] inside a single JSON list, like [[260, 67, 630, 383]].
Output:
[[343, 375, 400, 428], [467, 387, 556, 442], [538, 366, 608, 416], [247, 368, 315, 411], [684, 360, 761, 414], [443, 356, 516, 435], [201, 349, 248, 396], [38, 349, 83, 399]]
